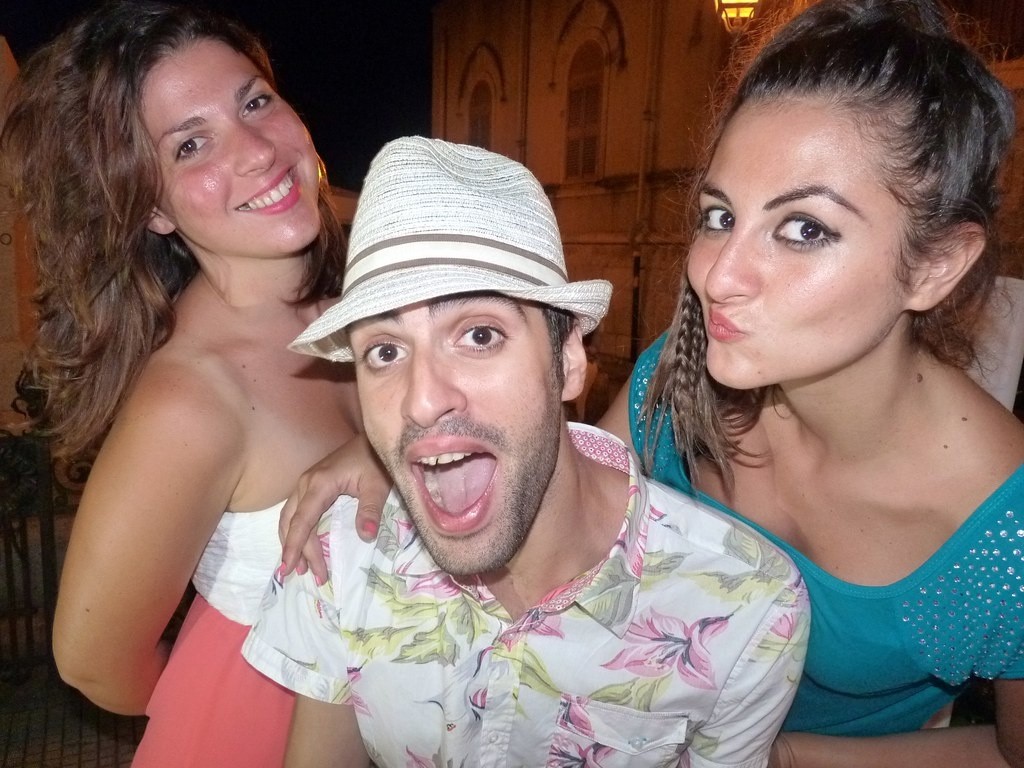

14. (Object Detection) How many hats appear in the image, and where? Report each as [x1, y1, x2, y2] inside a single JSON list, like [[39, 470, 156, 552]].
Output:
[[287, 135, 612, 362]]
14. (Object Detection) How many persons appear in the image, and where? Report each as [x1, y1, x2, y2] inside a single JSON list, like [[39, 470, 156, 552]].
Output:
[[0, 0, 365, 768], [277, 0, 1024, 768], [238, 135, 812, 768]]
